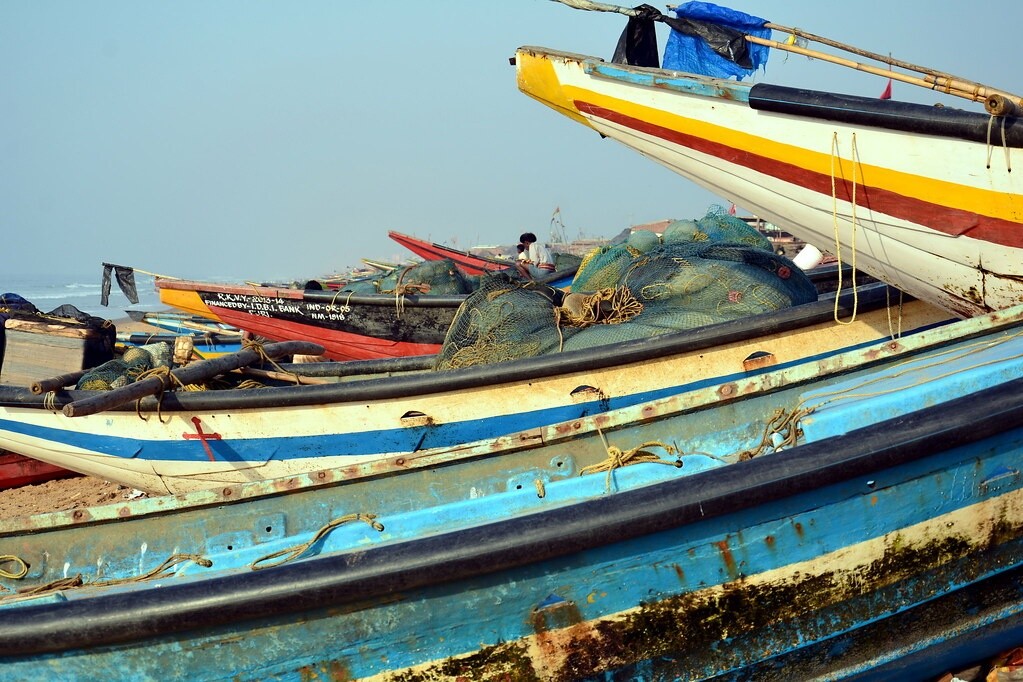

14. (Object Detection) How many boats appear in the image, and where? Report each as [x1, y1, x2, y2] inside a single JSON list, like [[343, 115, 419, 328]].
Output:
[[513, 42, 1022, 317], [2, 232, 1022, 682]]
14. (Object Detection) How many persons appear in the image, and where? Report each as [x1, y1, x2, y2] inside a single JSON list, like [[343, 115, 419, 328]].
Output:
[[515, 233, 555, 280]]
